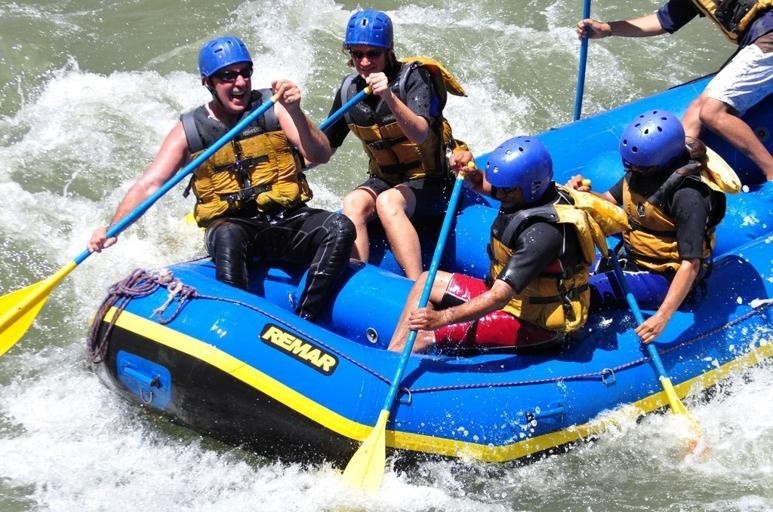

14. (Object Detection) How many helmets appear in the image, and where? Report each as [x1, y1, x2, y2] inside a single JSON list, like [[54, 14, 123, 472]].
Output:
[[618, 110, 686, 174], [344, 10, 394, 49], [198, 34, 253, 86], [485, 135, 554, 204]]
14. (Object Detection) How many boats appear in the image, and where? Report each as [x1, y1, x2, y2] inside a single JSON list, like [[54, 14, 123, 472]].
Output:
[[90, 67, 773, 483]]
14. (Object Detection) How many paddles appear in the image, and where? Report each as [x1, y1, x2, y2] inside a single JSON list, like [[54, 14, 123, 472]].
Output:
[[0, 93, 281, 357], [341, 169, 467, 494], [179, 85, 369, 235], [608, 249, 711, 463]]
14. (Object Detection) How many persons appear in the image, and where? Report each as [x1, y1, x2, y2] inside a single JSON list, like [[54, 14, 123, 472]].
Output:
[[84, 33, 355, 323], [561, 105, 722, 346], [298, 7, 454, 282], [574, 2, 772, 183], [385, 131, 595, 359]]
